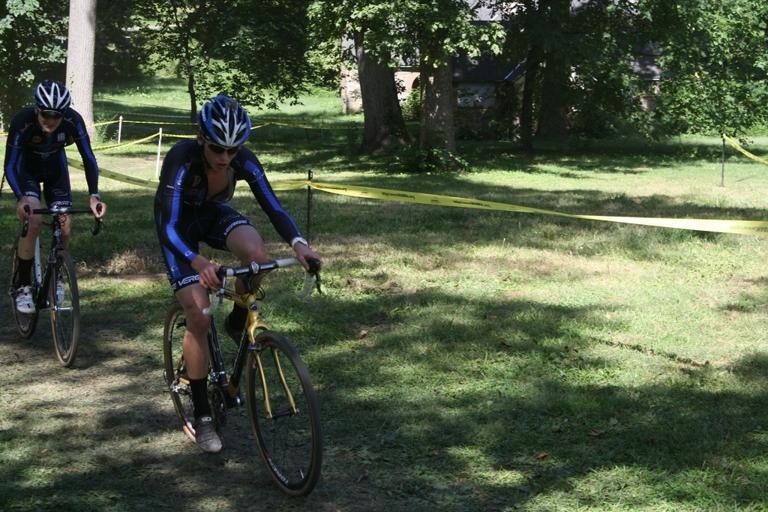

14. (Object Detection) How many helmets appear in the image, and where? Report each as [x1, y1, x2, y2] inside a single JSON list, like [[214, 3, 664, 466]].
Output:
[[36, 79, 71, 109], [200, 97, 252, 147]]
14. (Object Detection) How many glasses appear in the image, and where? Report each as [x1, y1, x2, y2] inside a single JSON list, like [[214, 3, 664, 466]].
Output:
[[203, 137, 238, 154], [39, 110, 63, 119]]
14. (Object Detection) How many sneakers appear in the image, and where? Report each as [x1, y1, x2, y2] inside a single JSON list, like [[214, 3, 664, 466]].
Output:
[[13, 284, 36, 314], [57, 280, 65, 302], [224, 315, 246, 348], [195, 419, 223, 454]]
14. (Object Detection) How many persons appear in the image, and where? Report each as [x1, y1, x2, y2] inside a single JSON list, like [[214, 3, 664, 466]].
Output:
[[151, 95, 320, 453], [3, 78, 107, 316]]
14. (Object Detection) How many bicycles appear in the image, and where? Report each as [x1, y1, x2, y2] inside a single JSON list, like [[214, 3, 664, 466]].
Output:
[[161, 255, 325, 498], [7, 201, 102, 368]]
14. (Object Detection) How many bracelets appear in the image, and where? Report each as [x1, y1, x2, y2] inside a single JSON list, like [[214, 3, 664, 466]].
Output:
[[90, 192, 101, 200]]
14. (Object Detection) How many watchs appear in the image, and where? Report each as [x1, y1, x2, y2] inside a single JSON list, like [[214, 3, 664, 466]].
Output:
[[289, 236, 309, 250]]
